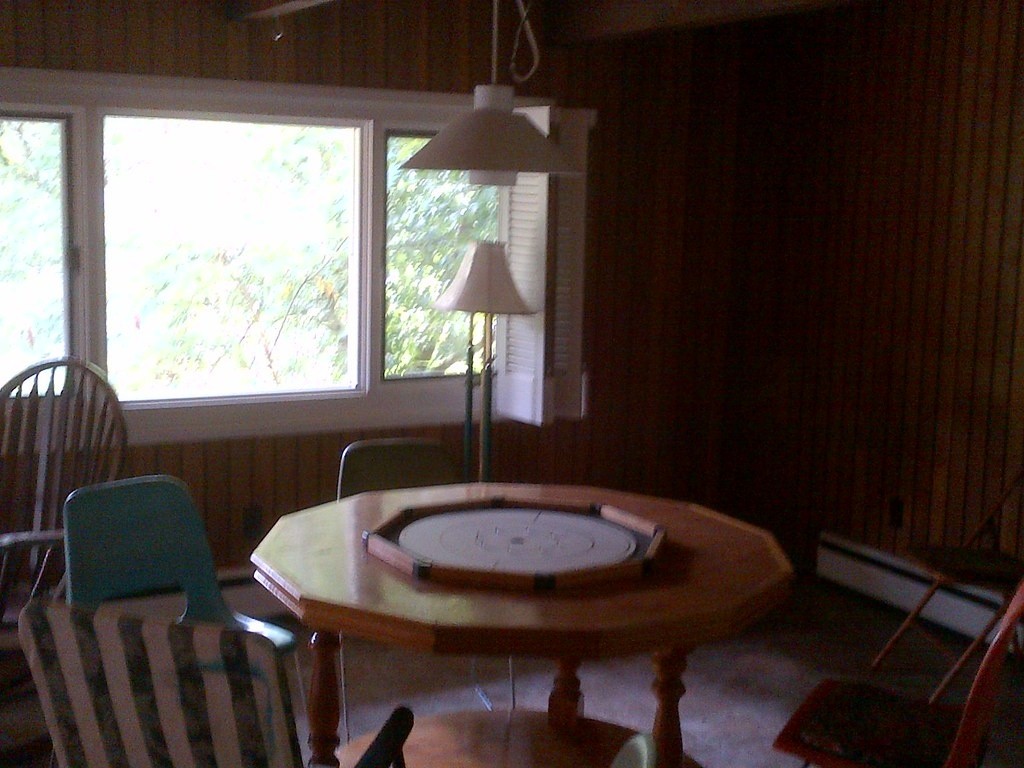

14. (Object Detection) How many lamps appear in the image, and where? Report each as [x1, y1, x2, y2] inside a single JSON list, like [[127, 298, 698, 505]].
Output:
[[430, 238, 535, 482], [397, 0, 588, 186]]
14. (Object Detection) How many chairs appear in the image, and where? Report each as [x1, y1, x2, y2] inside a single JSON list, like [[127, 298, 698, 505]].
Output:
[[17, 438, 517, 768], [0, 359, 129, 768], [774, 464, 1024, 768]]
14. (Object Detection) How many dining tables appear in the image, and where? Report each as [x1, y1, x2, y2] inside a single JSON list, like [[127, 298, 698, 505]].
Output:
[[252, 482, 793, 768]]
